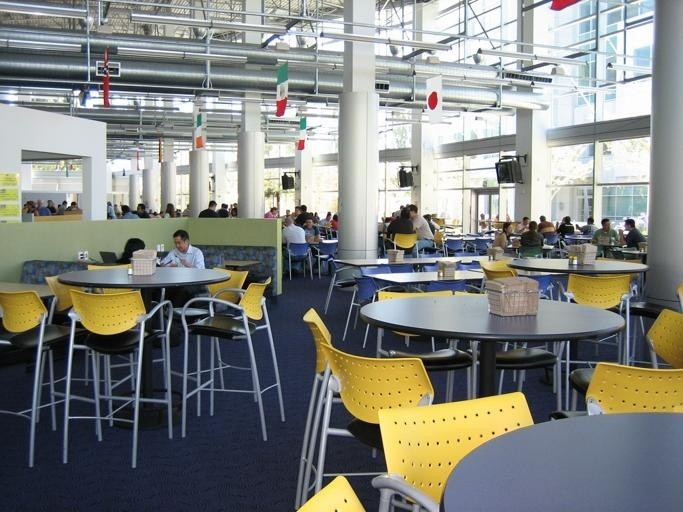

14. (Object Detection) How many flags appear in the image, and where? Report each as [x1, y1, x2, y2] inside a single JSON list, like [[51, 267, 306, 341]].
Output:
[[549, 0, 585, 10], [298, 117, 306, 151], [275, 62, 288, 116], [103, 46, 108, 106], [196, 113, 203, 150], [426, 75, 443, 124]]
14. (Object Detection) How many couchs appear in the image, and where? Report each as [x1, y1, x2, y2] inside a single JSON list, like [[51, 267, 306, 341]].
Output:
[[193, 245, 277, 306], [23, 260, 102, 325]]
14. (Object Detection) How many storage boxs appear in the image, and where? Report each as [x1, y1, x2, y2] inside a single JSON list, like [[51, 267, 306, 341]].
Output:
[[569, 243, 597, 267]]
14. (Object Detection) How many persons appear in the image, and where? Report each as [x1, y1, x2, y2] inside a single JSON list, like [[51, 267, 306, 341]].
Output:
[[619, 219, 646, 259], [66, 202, 79, 211], [36, 199, 52, 216], [282, 216, 307, 272], [163, 203, 174, 218], [290, 206, 301, 220], [390, 209, 402, 223], [330, 215, 338, 230], [423, 214, 435, 236], [577, 218, 596, 237], [303, 218, 322, 266], [120, 205, 140, 218], [218, 203, 229, 217], [265, 207, 278, 218], [46, 200, 58, 215], [174, 209, 183, 218], [229, 204, 234, 215], [295, 205, 318, 230], [148, 210, 164, 218], [59, 201, 68, 215], [26, 201, 40, 216], [517, 217, 530, 233], [108, 201, 117, 219], [520, 222, 544, 247], [387, 209, 416, 254], [161, 230, 209, 308], [479, 214, 488, 232], [199, 201, 218, 217], [556, 216, 574, 249], [493, 222, 513, 260], [537, 216, 558, 245], [114, 204, 121, 218], [285, 210, 290, 219], [22, 204, 29, 213], [408, 205, 434, 258], [314, 212, 321, 223], [114, 237, 145, 266], [136, 204, 151, 218], [319, 212, 332, 225], [592, 219, 620, 259], [231, 208, 238, 218]]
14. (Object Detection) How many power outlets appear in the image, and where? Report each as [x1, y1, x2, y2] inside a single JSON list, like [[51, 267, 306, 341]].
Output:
[[78, 252, 84, 260]]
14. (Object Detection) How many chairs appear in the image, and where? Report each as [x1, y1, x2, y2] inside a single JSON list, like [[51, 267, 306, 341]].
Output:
[[516, 246, 543, 259], [311, 241, 338, 279], [382, 233, 424, 272], [84, 264, 161, 393], [62, 289, 174, 468], [324, 259, 362, 315], [0, 291, 102, 468], [294, 308, 376, 512], [478, 259, 549, 350], [485, 242, 522, 261], [545, 232, 563, 258], [100, 252, 117, 263], [455, 252, 480, 257], [379, 264, 413, 272], [45, 272, 114, 430], [637, 242, 647, 251], [603, 245, 625, 259], [371, 393, 534, 512], [283, 243, 314, 281], [376, 290, 473, 403], [466, 238, 492, 254], [547, 362, 683, 419], [181, 277, 285, 442], [445, 239, 464, 257], [295, 476, 365, 512], [552, 274, 634, 411], [315, 343, 436, 491], [343, 275, 408, 349], [455, 291, 561, 399], [593, 257, 642, 366], [419, 232, 446, 258], [569, 309, 683, 411], [410, 279, 467, 354], [360, 265, 390, 276], [166, 267, 249, 389]]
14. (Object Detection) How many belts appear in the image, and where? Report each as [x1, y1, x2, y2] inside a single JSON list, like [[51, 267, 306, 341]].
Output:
[[417, 238, 433, 241]]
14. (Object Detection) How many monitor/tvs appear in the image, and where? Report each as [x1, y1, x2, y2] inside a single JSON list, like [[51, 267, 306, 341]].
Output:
[[282, 176, 294, 190], [495, 161, 523, 184], [397, 171, 414, 188]]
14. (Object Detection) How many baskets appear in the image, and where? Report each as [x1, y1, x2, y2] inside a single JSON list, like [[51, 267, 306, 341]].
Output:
[[566, 243, 597, 265], [437, 261, 455, 280], [387, 249, 405, 262], [130, 258, 156, 275]]
